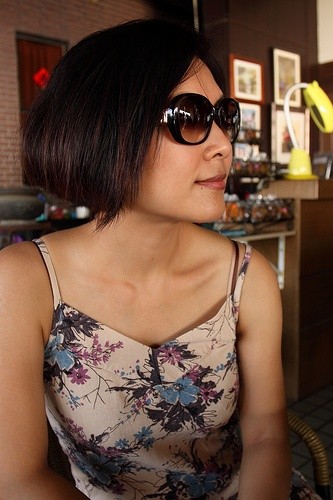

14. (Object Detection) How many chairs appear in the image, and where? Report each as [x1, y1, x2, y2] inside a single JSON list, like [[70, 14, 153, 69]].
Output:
[[285, 404, 332, 500]]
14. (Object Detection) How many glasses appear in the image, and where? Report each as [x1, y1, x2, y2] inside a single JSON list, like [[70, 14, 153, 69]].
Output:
[[155, 93, 240, 145]]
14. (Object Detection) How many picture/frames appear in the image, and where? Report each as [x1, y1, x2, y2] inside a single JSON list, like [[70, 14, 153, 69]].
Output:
[[269, 102, 310, 164], [229, 54, 265, 103], [231, 99, 265, 144], [270, 47, 304, 109]]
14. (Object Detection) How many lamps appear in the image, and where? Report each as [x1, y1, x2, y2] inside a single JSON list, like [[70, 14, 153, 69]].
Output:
[[283, 77, 333, 180]]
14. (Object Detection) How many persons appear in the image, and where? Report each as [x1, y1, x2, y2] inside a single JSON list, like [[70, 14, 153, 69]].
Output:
[[0, 18, 323, 500]]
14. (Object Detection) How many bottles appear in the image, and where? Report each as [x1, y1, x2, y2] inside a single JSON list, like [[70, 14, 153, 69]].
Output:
[[250, 131, 262, 163], [223, 192, 283, 222]]
